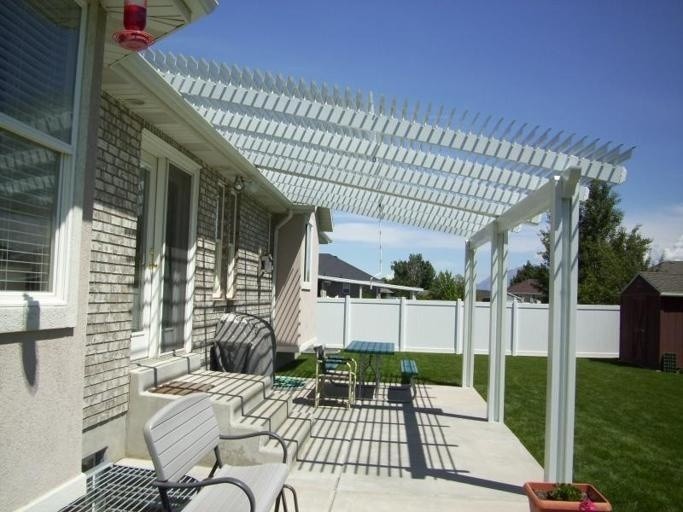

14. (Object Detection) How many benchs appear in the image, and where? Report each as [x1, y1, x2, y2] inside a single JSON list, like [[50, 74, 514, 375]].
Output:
[[401, 359, 419, 397], [322, 356, 343, 386]]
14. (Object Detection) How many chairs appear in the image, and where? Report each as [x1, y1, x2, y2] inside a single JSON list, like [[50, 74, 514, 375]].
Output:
[[315, 345, 357, 410], [143, 392, 298, 512]]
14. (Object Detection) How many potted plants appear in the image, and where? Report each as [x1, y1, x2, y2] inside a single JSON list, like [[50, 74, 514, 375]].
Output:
[[524, 481, 612, 512]]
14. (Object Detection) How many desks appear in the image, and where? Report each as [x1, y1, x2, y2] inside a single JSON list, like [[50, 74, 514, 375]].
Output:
[[344, 340, 394, 399]]
